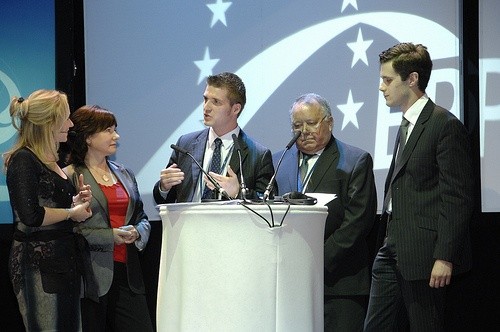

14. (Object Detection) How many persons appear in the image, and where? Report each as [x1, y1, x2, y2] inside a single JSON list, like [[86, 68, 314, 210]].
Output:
[[61, 103, 151, 332], [153, 72, 278, 205], [363, 42, 481, 332], [270, 93, 378, 332], [1, 88, 93, 332]]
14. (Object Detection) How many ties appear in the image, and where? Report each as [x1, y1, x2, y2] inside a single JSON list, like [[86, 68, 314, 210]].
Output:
[[300, 153, 313, 181], [379, 119, 411, 218], [201, 137, 222, 201]]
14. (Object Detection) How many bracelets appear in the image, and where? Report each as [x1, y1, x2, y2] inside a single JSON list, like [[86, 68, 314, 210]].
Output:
[[73, 195, 76, 207], [65, 208, 71, 222]]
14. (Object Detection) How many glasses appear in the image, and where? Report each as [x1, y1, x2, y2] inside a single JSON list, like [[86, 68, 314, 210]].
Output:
[[290, 113, 327, 132]]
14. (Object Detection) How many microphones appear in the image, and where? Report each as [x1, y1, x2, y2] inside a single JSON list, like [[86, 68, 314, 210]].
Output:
[[232, 133, 251, 205], [169, 143, 239, 204], [261, 130, 302, 205]]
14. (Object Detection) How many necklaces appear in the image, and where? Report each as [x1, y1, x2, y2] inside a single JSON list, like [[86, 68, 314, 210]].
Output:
[[84, 160, 109, 182]]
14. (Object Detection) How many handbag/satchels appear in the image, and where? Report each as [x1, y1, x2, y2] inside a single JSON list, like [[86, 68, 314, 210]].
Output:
[[38, 252, 86, 294]]
